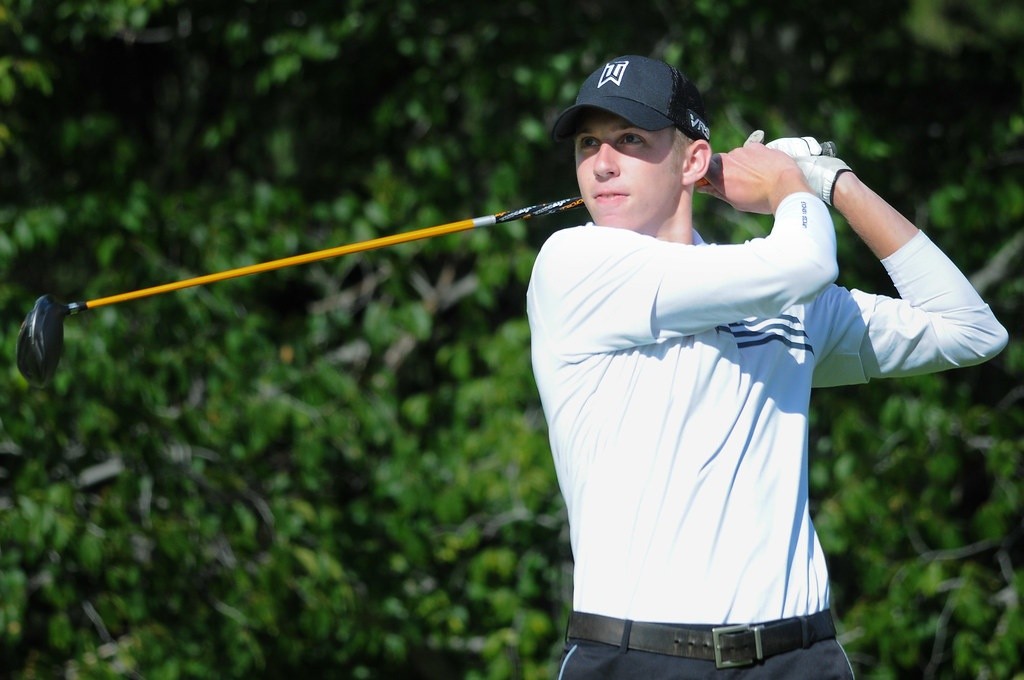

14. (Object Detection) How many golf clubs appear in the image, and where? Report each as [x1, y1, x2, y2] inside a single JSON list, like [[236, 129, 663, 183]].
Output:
[[14, 139, 841, 392]]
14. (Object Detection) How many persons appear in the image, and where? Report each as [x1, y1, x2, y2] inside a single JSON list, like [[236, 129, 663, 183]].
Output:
[[527, 52, 1011, 680]]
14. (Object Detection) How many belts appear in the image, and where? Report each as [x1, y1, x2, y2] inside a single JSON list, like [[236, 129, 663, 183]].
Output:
[[567, 608, 835, 669]]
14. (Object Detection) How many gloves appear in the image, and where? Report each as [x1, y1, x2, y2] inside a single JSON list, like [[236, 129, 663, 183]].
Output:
[[744, 129, 851, 207]]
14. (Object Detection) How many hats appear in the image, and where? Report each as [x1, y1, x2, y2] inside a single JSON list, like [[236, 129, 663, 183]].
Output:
[[551, 54, 710, 147]]
